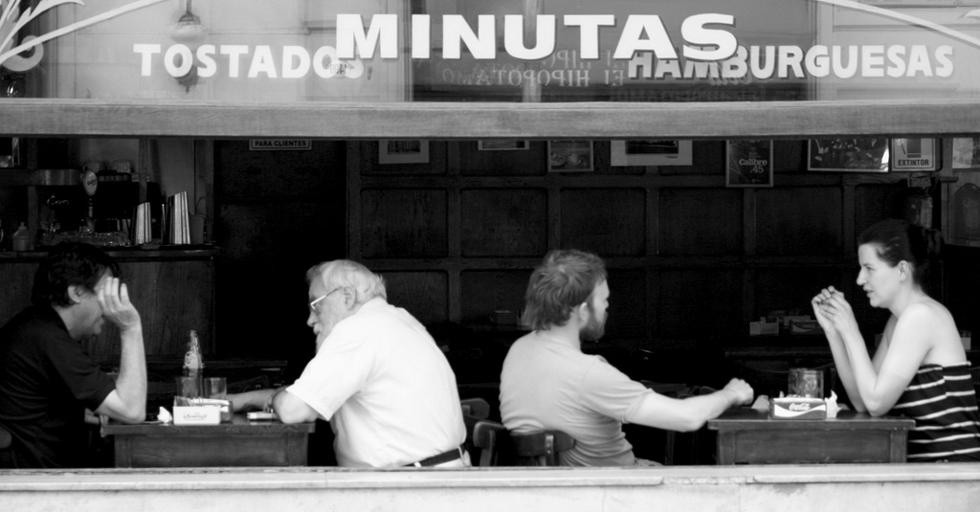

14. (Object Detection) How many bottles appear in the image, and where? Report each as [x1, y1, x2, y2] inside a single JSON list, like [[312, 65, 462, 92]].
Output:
[[12, 222, 31, 252]]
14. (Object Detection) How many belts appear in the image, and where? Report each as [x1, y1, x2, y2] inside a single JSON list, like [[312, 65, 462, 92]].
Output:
[[405, 444, 466, 467]]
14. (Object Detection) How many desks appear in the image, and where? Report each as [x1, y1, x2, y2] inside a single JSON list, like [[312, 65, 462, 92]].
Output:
[[101, 413, 315, 466], [708, 417, 915, 464]]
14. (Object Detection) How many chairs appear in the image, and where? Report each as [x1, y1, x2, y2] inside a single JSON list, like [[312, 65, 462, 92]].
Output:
[[459, 398, 578, 466]]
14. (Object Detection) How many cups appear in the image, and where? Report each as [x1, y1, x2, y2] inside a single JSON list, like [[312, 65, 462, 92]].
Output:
[[175, 377, 227, 399], [789, 367, 824, 399]]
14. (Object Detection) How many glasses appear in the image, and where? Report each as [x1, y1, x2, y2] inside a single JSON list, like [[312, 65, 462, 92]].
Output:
[[307, 286, 341, 312]]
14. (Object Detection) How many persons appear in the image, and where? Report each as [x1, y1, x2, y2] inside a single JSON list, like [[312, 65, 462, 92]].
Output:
[[497, 248, 754, 466], [212, 260, 473, 470], [812, 218, 979, 462], [0, 242, 149, 470]]
